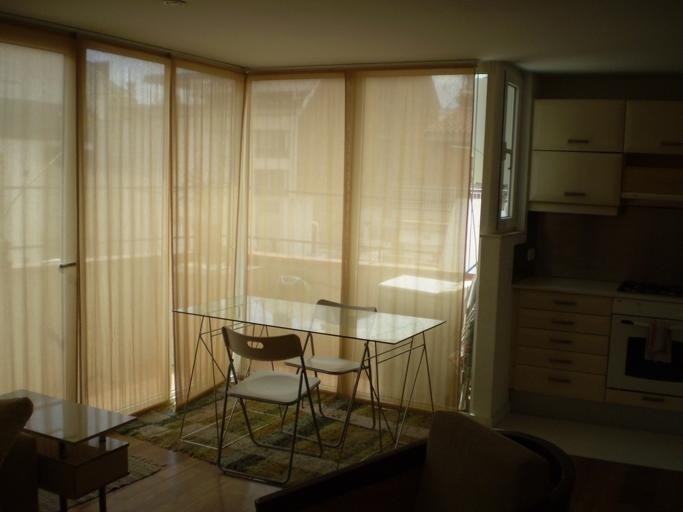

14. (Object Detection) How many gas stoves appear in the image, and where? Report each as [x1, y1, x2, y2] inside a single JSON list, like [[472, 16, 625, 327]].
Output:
[[614, 278, 682, 303]]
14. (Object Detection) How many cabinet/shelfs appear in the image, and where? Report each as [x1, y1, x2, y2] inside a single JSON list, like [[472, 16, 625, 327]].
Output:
[[511, 292, 613, 403], [624, 98, 682, 156], [527, 98, 626, 211]]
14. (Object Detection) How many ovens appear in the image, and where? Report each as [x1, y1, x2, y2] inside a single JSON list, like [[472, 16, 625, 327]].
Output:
[[604, 315, 682, 398]]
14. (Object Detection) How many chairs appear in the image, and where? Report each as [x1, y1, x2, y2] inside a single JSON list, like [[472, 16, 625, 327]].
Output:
[[278, 298, 378, 449], [214, 324, 323, 485]]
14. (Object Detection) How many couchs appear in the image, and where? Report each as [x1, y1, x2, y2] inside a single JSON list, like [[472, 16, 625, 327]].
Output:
[[252, 407, 682, 510], [0, 396, 40, 511]]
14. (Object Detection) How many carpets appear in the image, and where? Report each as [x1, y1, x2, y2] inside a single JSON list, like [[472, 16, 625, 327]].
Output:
[[107, 376, 440, 492], [35, 451, 164, 511]]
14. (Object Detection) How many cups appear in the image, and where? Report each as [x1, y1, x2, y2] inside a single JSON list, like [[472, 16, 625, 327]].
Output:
[[496, 216, 514, 235], [483, 214, 506, 236]]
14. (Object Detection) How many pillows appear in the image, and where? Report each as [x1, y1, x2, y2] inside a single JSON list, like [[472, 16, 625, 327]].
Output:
[[424, 409, 550, 509]]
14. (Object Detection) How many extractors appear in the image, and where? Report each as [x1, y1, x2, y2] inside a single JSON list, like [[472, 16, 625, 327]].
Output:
[[620, 192, 683, 208]]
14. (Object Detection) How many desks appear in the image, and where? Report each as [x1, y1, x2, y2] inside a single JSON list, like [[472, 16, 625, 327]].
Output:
[[0, 388, 137, 512], [169, 294, 448, 461]]
